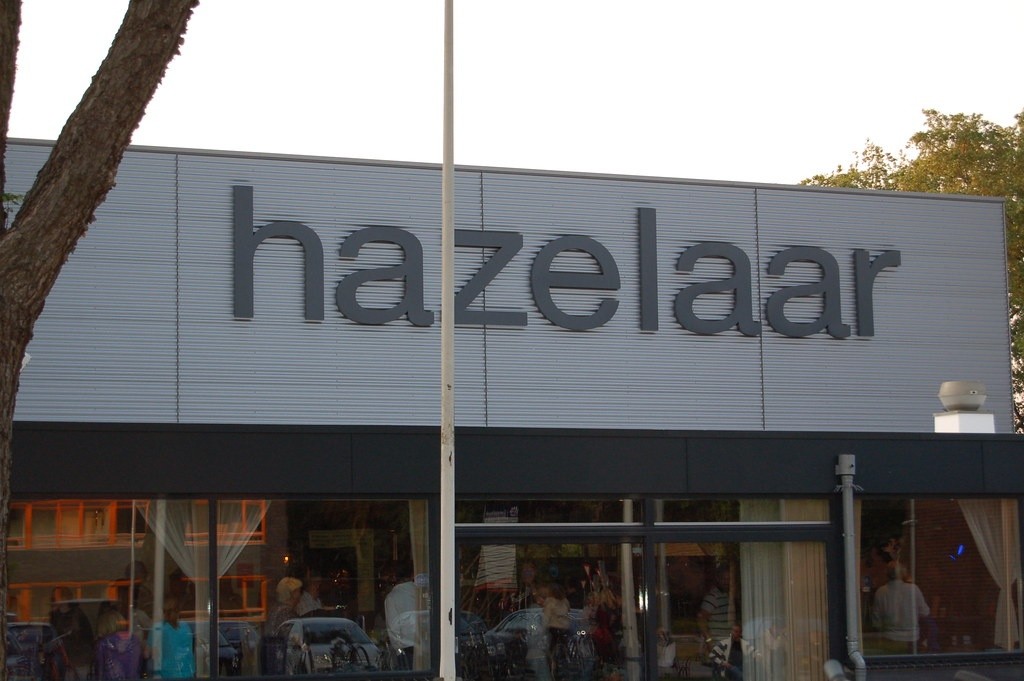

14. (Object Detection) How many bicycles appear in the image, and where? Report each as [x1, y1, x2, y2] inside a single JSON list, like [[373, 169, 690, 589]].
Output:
[[460, 626, 599, 681]]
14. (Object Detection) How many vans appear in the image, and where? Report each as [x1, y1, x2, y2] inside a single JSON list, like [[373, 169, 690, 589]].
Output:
[[50, 599, 147, 666]]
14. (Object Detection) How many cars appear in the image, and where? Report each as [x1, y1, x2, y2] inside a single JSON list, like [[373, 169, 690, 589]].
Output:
[[185, 618, 259, 678], [483, 607, 587, 657], [265, 618, 382, 675], [6, 621, 57, 680], [381, 611, 480, 671]]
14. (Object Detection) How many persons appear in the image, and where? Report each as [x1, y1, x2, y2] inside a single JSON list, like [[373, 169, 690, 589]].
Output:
[[261, 568, 323, 675], [95, 608, 151, 681], [382, 561, 416, 670], [709, 624, 760, 681], [868, 561, 929, 656], [108, 561, 153, 619], [697, 563, 742, 681], [543, 581, 622, 681], [39, 585, 94, 681], [143, 593, 194, 681]]
